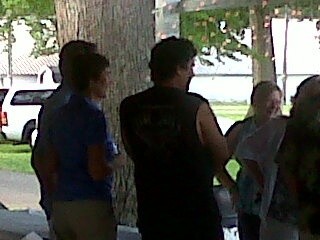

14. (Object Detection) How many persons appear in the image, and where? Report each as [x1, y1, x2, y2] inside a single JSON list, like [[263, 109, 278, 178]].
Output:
[[234, 73, 320, 240], [31, 39, 98, 240], [119, 35, 231, 240], [35, 51, 128, 240], [216, 80, 283, 240]]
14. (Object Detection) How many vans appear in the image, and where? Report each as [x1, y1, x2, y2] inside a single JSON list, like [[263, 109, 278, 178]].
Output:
[[1, 82, 66, 143]]
